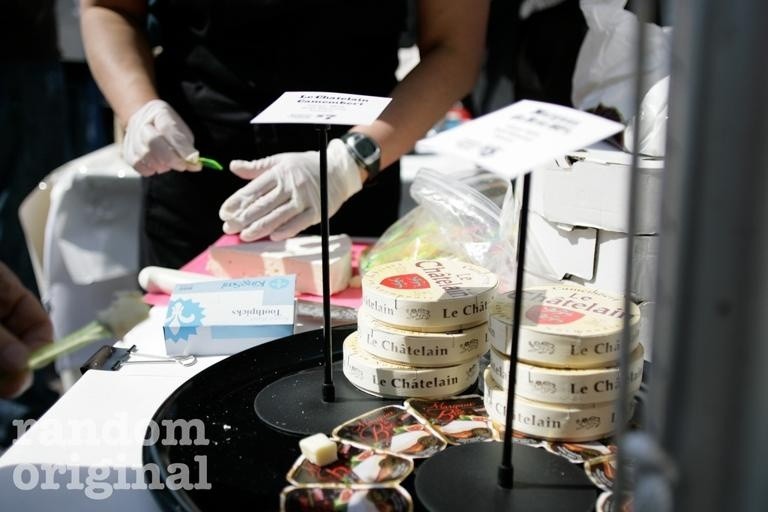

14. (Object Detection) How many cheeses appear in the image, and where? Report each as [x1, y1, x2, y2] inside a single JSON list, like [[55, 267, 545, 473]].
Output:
[[299, 434, 339, 465], [206, 233, 353, 296]]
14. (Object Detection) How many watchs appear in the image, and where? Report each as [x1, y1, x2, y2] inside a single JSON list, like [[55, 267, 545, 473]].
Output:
[[340, 131, 382, 181]]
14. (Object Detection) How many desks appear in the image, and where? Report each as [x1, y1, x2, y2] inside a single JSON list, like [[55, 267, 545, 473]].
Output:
[[0, 233, 644, 511]]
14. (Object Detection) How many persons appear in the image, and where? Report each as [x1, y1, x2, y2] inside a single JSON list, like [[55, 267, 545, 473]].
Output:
[[0, 262, 56, 400], [73, 2, 493, 303]]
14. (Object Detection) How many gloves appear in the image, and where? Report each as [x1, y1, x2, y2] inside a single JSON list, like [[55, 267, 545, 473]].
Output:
[[120, 99, 203, 178], [218, 137, 364, 243]]
